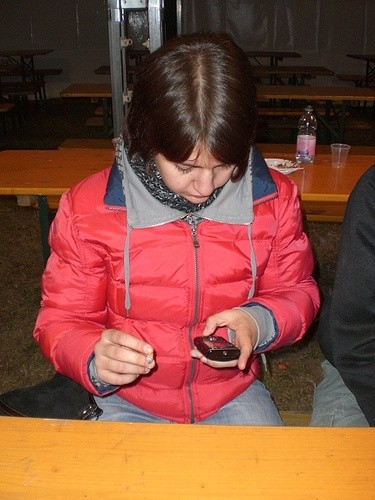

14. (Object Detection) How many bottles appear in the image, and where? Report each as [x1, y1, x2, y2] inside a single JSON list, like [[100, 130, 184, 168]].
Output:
[[295, 105, 318, 164]]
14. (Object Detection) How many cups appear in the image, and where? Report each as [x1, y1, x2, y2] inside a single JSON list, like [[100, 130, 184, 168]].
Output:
[[330, 144, 350, 169]]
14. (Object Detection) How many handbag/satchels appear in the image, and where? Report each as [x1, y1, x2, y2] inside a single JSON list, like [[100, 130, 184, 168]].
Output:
[[0, 374, 103, 420]]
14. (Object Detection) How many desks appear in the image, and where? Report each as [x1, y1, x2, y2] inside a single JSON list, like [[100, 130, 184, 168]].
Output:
[[60, 82, 136, 138], [252, 85, 375, 141], [252, 144, 375, 203], [0, 48, 53, 115], [127, 49, 150, 66], [245, 51, 301, 85], [0, 411, 375, 500], [346, 54, 375, 115], [95, 66, 142, 84], [0, 80, 47, 130], [0, 147, 117, 267], [249, 66, 333, 83]]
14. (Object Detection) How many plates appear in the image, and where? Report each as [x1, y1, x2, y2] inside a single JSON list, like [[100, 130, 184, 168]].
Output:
[[264, 158, 300, 175]]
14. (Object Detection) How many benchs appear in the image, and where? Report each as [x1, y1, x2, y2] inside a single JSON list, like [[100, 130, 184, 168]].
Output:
[[0, 70, 375, 222]]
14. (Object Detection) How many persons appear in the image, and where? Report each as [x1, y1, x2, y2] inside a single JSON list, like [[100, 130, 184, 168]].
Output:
[[308, 165, 375, 428], [32, 32, 321, 426]]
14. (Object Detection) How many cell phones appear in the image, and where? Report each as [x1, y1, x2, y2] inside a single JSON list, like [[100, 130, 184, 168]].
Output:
[[194, 335, 240, 361]]
